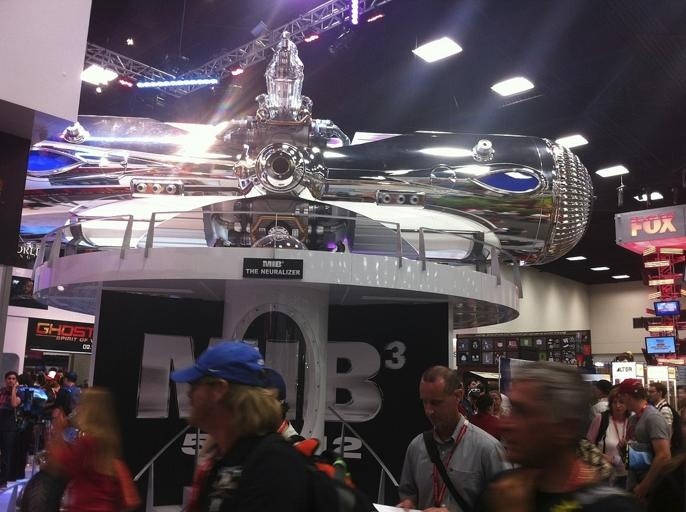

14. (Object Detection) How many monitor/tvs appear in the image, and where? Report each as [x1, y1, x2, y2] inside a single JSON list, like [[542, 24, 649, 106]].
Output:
[[644, 336, 677, 356], [654, 301, 680, 316]]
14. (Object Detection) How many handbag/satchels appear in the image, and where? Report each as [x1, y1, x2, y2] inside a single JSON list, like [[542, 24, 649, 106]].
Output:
[[576, 437, 612, 480], [624, 440, 654, 472], [660, 405, 681, 451]]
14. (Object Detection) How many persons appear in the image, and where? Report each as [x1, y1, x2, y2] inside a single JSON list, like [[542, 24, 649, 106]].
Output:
[[1, 337, 685, 512]]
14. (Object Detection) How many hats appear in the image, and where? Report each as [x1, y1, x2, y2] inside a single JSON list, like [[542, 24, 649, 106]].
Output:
[[169, 341, 264, 386], [619, 379, 641, 393]]
